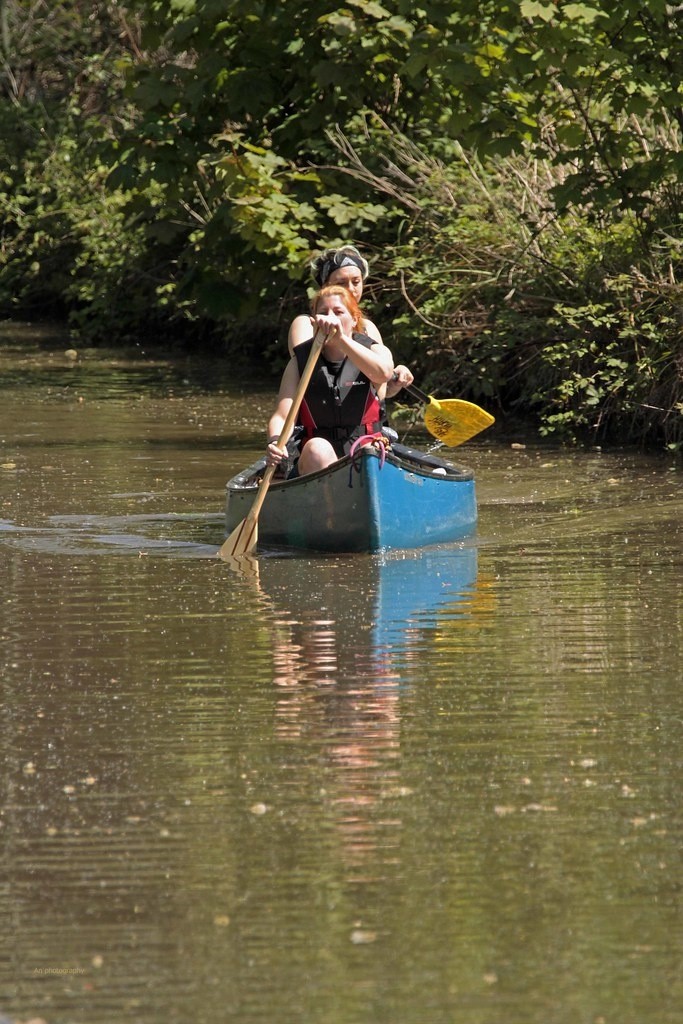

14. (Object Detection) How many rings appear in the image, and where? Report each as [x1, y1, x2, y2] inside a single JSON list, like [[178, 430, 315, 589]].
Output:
[[269, 452, 272, 455], [404, 379, 408, 383]]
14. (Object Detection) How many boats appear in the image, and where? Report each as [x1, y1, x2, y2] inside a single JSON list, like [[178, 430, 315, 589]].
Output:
[[253, 551, 477, 664], [226, 427, 476, 554]]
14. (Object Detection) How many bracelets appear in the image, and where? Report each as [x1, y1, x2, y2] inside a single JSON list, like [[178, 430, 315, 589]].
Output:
[[267, 435, 281, 446]]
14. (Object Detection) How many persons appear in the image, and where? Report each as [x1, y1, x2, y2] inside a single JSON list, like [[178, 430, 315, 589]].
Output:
[[266, 244, 414, 479]]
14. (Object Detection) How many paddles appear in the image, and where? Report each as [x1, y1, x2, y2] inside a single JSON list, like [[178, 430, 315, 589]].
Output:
[[213, 323, 337, 561], [388, 372, 496, 453]]
